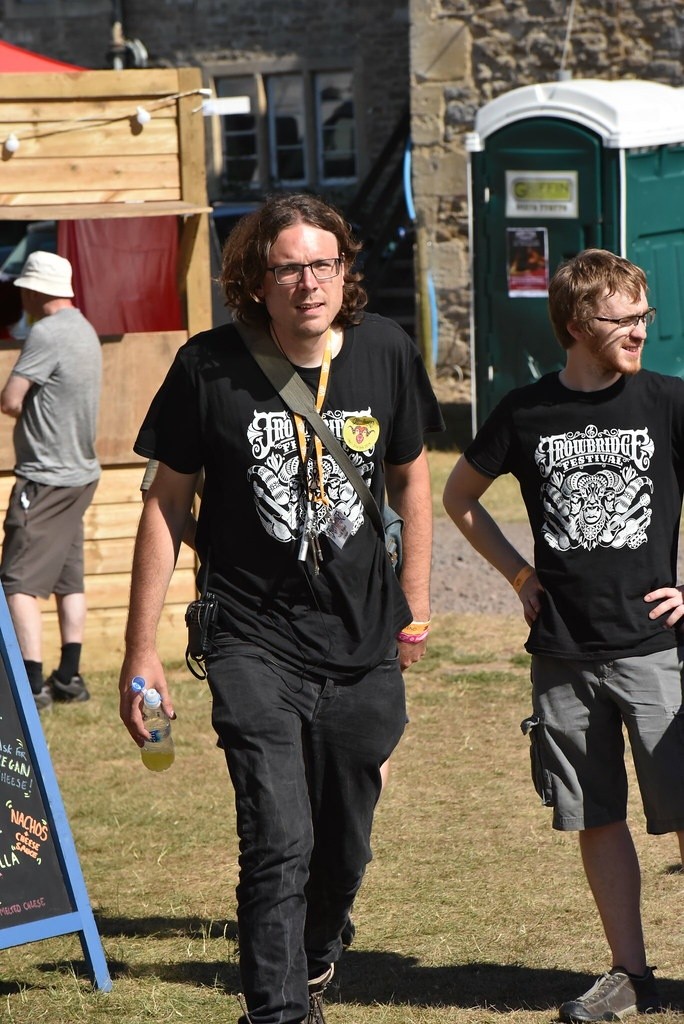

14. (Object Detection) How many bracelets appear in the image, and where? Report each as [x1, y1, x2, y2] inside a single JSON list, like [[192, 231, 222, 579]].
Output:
[[512, 565, 534, 594], [398, 619, 431, 643]]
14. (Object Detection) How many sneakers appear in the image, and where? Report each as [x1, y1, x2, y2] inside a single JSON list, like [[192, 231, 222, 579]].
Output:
[[33, 685, 54, 716], [44, 670, 91, 702], [559, 965, 657, 1021]]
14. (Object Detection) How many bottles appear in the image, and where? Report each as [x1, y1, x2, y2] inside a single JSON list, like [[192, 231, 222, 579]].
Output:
[[131, 676, 175, 772]]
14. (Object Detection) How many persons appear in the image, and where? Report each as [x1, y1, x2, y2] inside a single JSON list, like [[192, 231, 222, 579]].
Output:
[[441, 249, 684, 1024], [0, 251, 102, 715], [118, 193, 448, 1024]]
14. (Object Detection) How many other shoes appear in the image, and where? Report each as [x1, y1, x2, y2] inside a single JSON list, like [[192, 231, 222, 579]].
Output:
[[306, 962, 335, 1024]]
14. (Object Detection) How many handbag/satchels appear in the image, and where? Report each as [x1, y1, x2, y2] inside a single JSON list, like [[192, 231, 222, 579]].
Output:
[[384, 507, 406, 580]]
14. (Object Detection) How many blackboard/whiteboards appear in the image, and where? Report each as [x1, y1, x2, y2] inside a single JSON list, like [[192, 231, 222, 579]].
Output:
[[0, 580, 97, 950]]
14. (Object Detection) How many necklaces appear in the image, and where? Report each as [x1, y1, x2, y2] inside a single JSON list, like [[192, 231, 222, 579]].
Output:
[[271, 321, 288, 359]]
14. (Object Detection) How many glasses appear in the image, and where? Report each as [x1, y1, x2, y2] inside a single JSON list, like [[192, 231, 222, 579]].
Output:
[[591, 307, 656, 332], [267, 256, 344, 285]]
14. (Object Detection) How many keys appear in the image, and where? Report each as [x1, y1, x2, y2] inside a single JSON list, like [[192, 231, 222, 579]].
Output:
[[308, 529, 323, 576]]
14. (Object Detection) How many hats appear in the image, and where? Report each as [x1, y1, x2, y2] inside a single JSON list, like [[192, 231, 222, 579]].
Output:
[[13, 251, 75, 298]]
